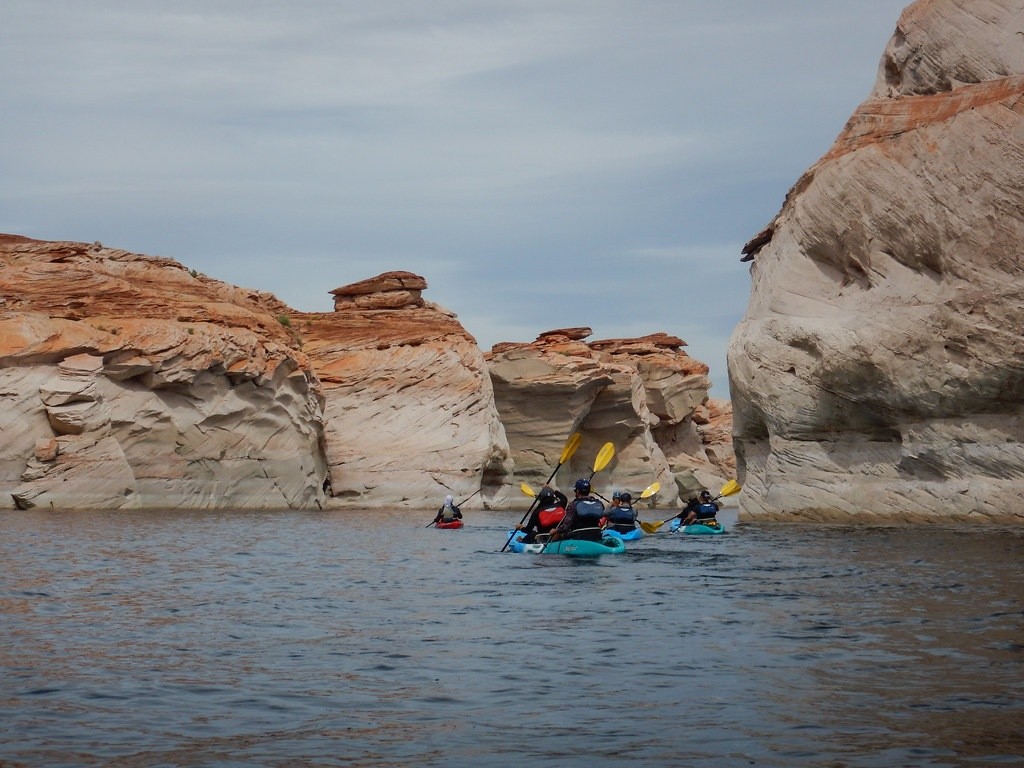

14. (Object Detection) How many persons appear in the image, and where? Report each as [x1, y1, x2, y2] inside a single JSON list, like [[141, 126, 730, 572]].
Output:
[[676, 494, 700, 524], [516, 483, 567, 544], [605, 492, 621, 510], [686, 490, 719, 525], [550, 479, 605, 541], [434, 494, 463, 523], [603, 493, 638, 534]]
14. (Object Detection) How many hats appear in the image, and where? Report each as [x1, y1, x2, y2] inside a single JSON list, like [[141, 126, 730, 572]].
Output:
[[535, 486, 557, 500], [688, 498, 700, 506], [574, 479, 591, 492], [701, 491, 710, 500], [613, 492, 621, 498], [621, 493, 632, 501]]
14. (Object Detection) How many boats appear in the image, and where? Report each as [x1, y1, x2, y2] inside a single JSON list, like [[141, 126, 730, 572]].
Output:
[[670, 518, 725, 536], [506, 530, 626, 557], [435, 520, 465, 530], [599, 525, 644, 542]]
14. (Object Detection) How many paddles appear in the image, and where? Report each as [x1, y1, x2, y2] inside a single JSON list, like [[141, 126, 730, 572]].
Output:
[[588, 485, 657, 534], [654, 479, 743, 534], [520, 483, 545, 504], [425, 487, 486, 528], [500, 432, 583, 552], [630, 482, 660, 505], [540, 442, 614, 553]]
